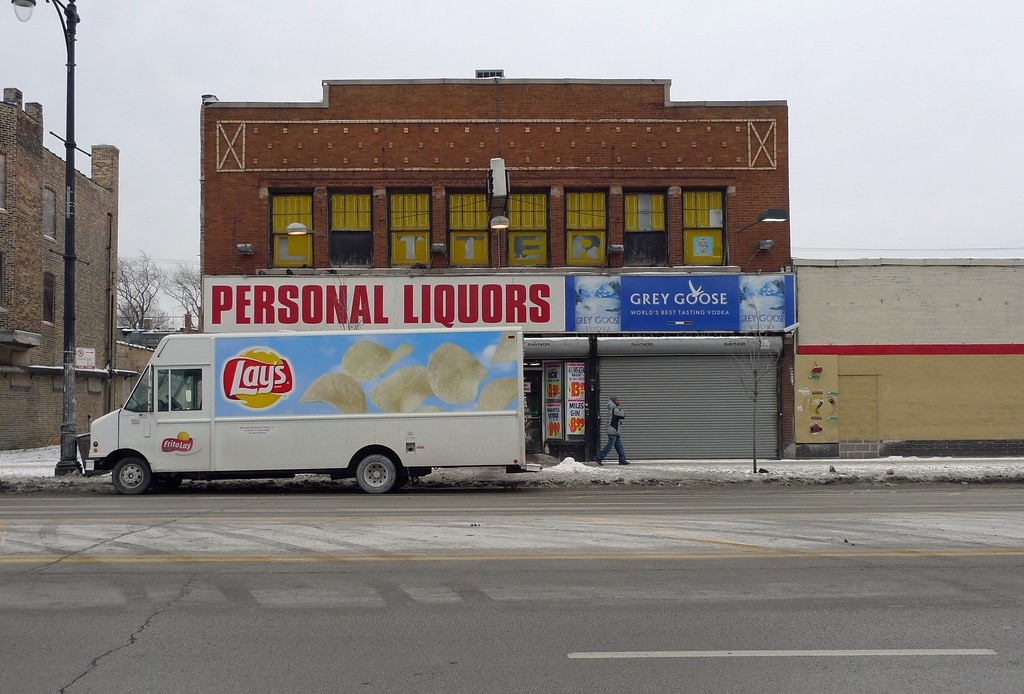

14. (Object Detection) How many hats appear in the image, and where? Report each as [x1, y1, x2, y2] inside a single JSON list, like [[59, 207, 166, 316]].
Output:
[[610, 395, 616, 400]]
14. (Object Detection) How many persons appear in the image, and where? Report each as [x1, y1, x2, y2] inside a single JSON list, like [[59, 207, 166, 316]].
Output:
[[594, 396, 630, 465]]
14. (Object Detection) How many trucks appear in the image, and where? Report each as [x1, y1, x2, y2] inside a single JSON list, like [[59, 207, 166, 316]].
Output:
[[86, 324, 527, 495]]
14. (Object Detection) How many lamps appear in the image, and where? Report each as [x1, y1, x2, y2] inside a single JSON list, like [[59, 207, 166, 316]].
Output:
[[610, 244, 624, 254], [286, 222, 324, 237], [757, 239, 776, 250], [430, 243, 446, 253], [491, 216, 509, 228], [737, 208, 787, 231], [238, 244, 255, 254]]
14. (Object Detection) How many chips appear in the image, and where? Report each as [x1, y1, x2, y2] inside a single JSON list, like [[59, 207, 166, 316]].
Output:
[[298, 331, 521, 412]]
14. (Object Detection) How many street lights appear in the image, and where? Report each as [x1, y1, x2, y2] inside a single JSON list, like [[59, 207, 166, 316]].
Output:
[[8, 0, 92, 476]]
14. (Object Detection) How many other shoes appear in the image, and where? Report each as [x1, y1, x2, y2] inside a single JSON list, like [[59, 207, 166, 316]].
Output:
[[593, 457, 603, 466], [619, 462, 630, 464]]
